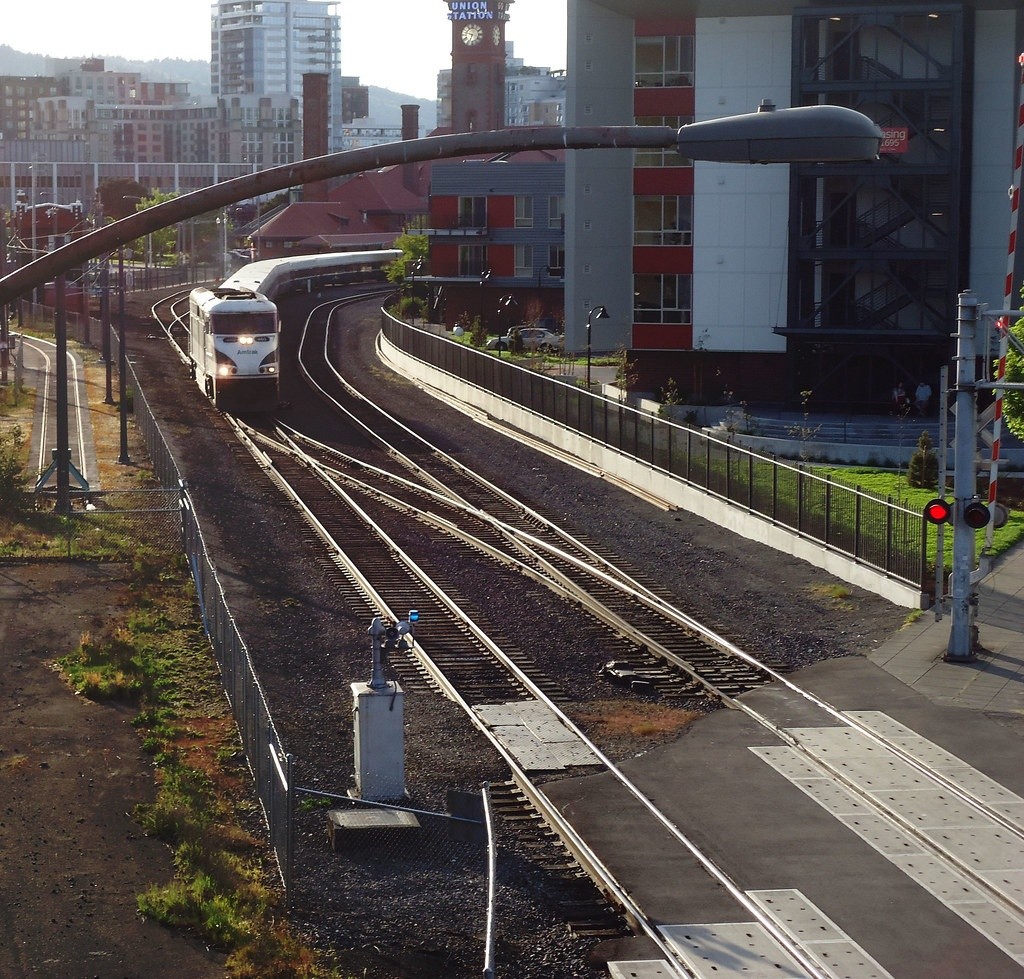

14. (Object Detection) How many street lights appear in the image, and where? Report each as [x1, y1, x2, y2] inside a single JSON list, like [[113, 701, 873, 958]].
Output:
[[497, 294, 519, 356], [586, 305, 610, 387], [480, 268, 492, 345], [411, 255, 425, 326]]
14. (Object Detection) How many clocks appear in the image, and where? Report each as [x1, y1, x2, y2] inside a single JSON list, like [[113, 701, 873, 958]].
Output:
[[492, 23, 500, 46], [461, 23, 483, 46]]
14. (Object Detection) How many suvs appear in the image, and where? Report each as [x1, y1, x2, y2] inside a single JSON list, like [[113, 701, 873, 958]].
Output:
[[506, 318, 561, 336]]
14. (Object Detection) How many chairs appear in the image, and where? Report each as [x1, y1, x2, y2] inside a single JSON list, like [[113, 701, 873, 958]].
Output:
[[670, 219, 691, 245]]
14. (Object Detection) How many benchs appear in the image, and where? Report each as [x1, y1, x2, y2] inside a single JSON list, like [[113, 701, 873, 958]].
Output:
[[767, 402, 939, 423]]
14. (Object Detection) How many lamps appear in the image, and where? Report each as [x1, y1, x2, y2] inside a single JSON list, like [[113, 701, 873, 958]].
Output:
[[634, 289, 640, 296]]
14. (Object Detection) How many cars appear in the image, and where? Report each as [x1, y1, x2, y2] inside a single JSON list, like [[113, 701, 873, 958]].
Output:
[[485, 328, 564, 352]]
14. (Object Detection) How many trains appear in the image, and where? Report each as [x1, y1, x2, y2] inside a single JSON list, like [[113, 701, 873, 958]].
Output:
[[188, 249, 403, 406]]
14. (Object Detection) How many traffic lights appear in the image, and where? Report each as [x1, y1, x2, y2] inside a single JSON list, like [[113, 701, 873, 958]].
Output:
[[925, 499, 951, 524], [966, 502, 991, 528]]
